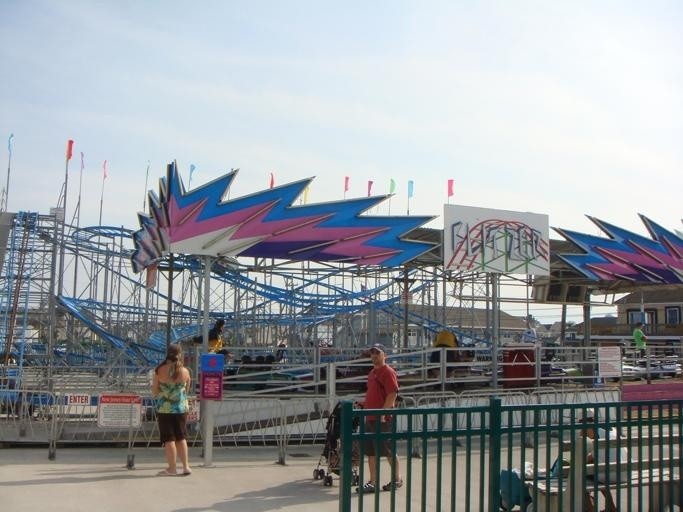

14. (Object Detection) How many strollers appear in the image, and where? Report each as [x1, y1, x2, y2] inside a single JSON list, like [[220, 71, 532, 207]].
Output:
[[500, 453, 570, 512], [313, 400, 364, 486]]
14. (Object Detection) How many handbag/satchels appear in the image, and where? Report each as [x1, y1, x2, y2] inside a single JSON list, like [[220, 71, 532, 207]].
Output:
[[186, 396, 199, 424]]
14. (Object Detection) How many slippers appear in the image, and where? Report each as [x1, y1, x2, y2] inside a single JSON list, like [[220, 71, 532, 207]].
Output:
[[182, 466, 194, 474], [156, 470, 177, 477]]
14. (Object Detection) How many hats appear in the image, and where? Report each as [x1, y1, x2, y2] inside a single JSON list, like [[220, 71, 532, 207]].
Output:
[[369, 343, 386, 352]]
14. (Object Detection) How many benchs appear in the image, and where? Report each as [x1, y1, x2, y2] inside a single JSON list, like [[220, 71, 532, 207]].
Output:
[[511, 434, 680, 510]]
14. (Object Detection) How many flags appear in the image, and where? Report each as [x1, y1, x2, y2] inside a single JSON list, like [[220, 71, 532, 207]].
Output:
[[66, 139, 74, 160], [345, 176, 350, 191], [448, 179, 454, 196], [189, 164, 196, 180], [270, 176, 274, 187], [8, 134, 15, 154], [390, 179, 396, 193], [368, 181, 373, 196], [408, 181, 414, 197], [304, 186, 309, 203]]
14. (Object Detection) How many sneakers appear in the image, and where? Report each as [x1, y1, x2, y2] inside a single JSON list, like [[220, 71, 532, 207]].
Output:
[[383, 478, 404, 491], [356, 481, 376, 494]]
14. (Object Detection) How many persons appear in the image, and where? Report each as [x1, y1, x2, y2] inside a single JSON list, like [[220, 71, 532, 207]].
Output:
[[522, 322, 536, 343], [355, 343, 403, 492], [152, 344, 192, 476], [578, 418, 627, 512], [209, 319, 226, 353], [431, 330, 458, 378], [237, 355, 275, 374], [633, 322, 647, 358]]
